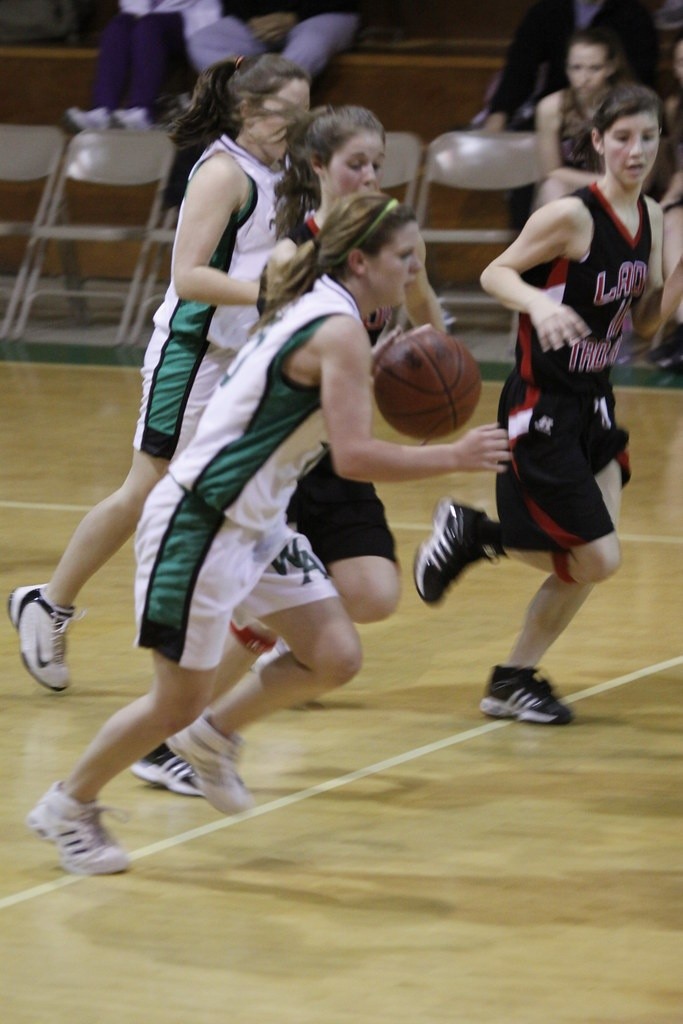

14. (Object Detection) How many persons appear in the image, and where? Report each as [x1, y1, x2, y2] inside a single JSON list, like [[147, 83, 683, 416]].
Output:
[[24, 192, 510, 877], [9, 0, 683, 798]]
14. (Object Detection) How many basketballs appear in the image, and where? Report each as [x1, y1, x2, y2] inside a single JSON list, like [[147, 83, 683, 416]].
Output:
[[372, 328, 481, 439]]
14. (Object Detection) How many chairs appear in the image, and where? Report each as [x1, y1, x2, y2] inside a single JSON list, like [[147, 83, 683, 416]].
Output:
[[1, 116, 555, 379]]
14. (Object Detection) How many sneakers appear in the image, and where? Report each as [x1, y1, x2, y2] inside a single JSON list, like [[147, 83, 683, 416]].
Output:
[[24, 780, 130, 876], [413, 497, 488, 603], [129, 741, 206, 798], [114, 108, 150, 132], [66, 106, 109, 133], [8, 582, 88, 691], [165, 715, 254, 815], [479, 665, 573, 726]]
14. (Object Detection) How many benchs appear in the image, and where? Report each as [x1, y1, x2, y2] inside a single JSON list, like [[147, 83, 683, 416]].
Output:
[[2, 11, 568, 73]]
